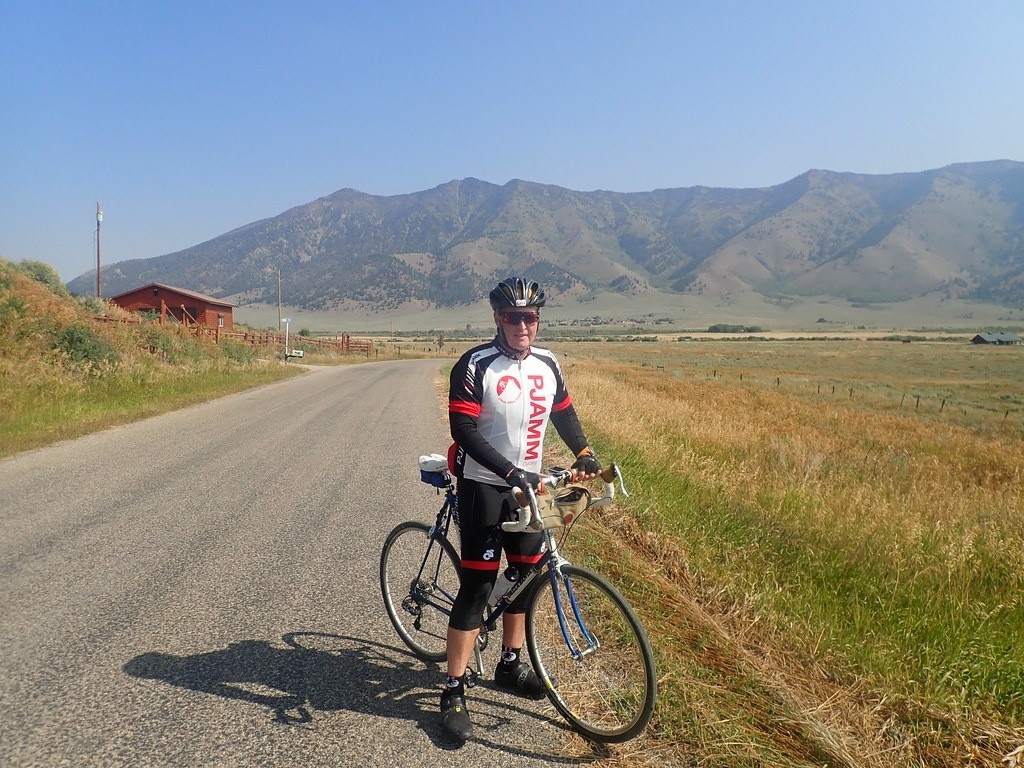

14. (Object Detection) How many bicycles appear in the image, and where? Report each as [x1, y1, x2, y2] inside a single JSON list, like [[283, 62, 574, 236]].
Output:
[[381, 449, 658, 748]]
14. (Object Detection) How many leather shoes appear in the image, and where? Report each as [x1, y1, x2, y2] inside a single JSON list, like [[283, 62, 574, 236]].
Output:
[[495, 662, 558, 693], [440, 690, 473, 741]]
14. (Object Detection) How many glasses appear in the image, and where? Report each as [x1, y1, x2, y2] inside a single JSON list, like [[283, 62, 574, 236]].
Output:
[[495, 312, 541, 325]]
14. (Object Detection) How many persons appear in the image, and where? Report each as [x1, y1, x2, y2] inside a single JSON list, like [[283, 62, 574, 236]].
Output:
[[439, 277, 603, 738]]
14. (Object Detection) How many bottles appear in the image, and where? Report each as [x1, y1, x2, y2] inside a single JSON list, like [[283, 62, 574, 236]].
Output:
[[487, 566, 520, 607]]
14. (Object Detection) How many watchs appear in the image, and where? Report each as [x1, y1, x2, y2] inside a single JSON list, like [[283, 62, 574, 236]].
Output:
[[578, 449, 595, 458]]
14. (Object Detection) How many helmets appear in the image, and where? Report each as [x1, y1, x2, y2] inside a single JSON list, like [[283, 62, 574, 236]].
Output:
[[490, 277, 546, 310]]
